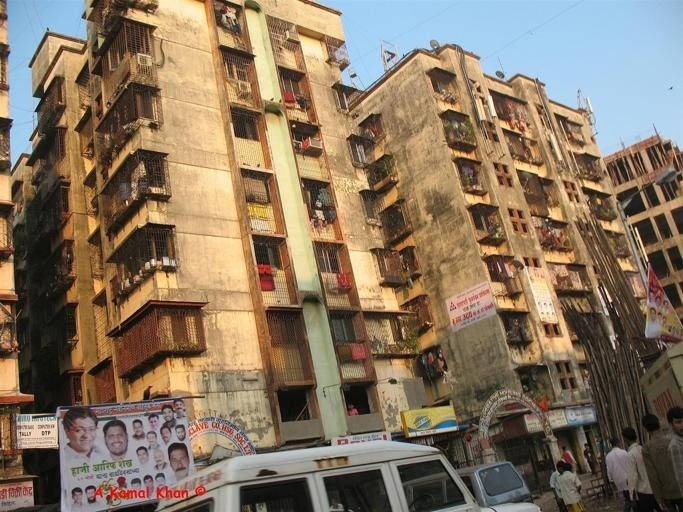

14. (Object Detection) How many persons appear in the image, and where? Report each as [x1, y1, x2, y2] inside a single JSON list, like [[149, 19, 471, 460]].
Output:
[[664, 406, 682, 496], [639, 414, 683, 511], [620, 426, 661, 511], [604, 436, 639, 511], [347, 402, 359, 416], [560, 446, 578, 475], [558, 463, 587, 512], [645, 288, 667, 338], [61, 398, 188, 512], [582, 443, 599, 476], [328, 498, 346, 510], [549, 461, 568, 512]]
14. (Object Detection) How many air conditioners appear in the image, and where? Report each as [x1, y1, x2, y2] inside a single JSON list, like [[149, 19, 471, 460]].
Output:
[[284, 31, 299, 41], [236, 80, 251, 95], [302, 135, 324, 153], [135, 53, 153, 68]]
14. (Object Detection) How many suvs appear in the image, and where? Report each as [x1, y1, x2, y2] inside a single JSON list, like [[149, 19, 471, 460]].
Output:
[[152, 440, 541, 512]]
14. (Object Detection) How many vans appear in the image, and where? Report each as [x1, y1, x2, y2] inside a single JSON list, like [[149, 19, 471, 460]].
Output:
[[376, 460, 535, 512]]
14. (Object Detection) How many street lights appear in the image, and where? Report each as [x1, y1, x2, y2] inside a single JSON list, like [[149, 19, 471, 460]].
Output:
[[614, 166, 676, 295]]
